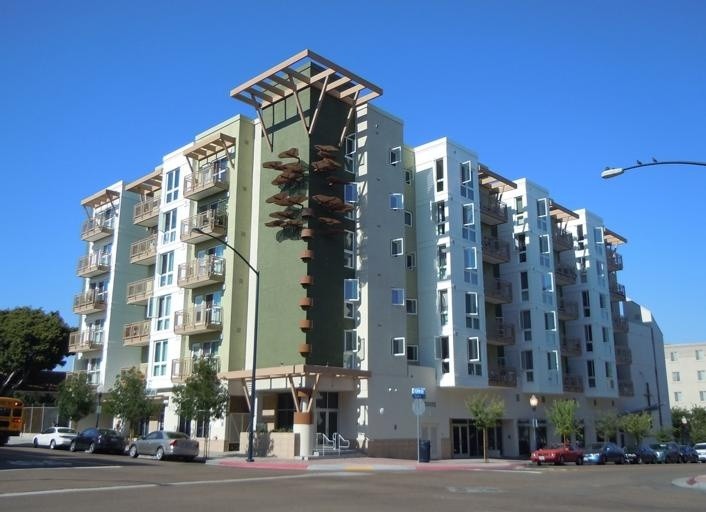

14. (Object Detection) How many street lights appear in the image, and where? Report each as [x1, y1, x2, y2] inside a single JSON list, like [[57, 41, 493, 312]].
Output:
[[600, 157, 705, 178], [529, 394, 540, 450], [190, 227, 259, 464], [680, 415, 687, 445]]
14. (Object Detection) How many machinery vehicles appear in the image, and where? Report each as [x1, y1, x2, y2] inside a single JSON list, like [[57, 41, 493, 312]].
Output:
[[0, 370, 24, 445]]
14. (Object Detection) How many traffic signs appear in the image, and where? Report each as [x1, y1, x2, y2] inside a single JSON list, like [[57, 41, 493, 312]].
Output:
[[412, 394, 425, 399]]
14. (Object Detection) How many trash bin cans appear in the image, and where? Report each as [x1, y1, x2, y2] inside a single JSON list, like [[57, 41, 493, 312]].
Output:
[[419, 440, 430, 462]]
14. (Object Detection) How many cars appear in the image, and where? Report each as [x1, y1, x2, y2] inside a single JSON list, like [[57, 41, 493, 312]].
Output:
[[529, 442, 706, 466], [68, 428, 125, 455], [128, 431, 198, 461], [31, 427, 79, 450]]
